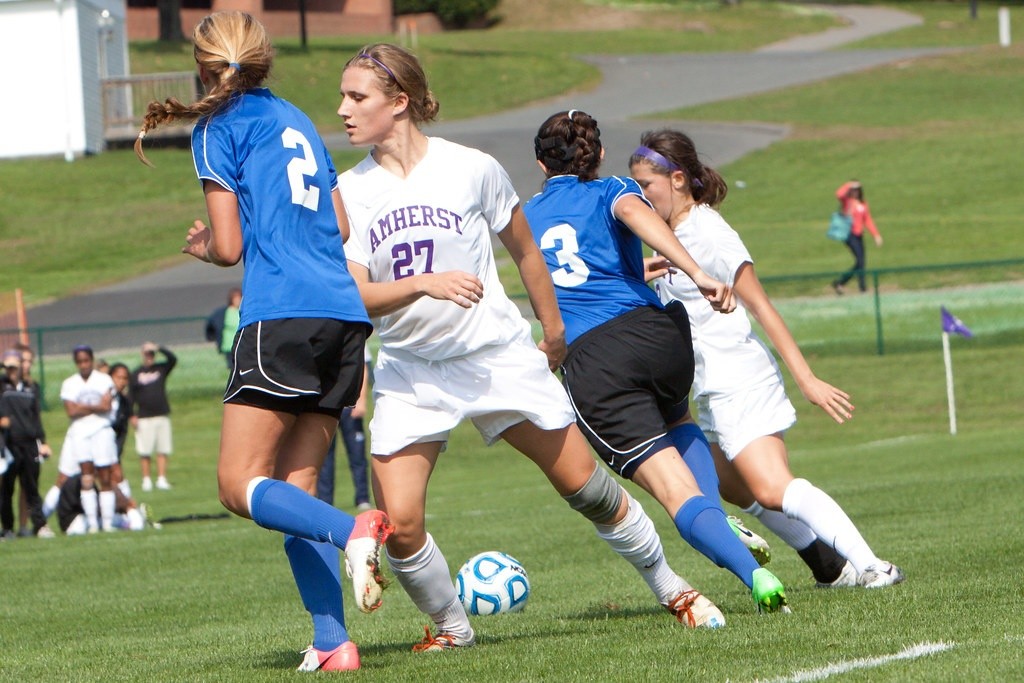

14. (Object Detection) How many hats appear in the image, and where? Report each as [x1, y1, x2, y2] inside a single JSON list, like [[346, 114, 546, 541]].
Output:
[[3, 349, 22, 369]]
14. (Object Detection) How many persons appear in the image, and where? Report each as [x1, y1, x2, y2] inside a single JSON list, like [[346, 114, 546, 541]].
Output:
[[520, 108, 790, 614], [95, 359, 109, 373], [205, 289, 242, 370], [42, 362, 131, 518], [130, 341, 176, 492], [336, 41, 726, 650], [133, 10, 391, 672], [59, 473, 144, 532], [629, 126, 902, 587], [316, 341, 373, 508], [60, 346, 121, 535], [830, 178, 883, 297], [0, 343, 57, 538]]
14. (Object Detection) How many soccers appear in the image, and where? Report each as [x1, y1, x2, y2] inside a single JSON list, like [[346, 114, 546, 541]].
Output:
[[454, 550, 530, 615]]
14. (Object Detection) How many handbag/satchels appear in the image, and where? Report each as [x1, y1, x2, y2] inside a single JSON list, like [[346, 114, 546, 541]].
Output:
[[825, 213, 853, 241]]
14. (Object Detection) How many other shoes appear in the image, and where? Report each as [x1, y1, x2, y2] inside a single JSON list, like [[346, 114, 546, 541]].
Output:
[[37, 525, 56, 539], [17, 527, 31, 538], [129, 514, 144, 531], [814, 559, 858, 589], [102, 524, 116, 533], [156, 477, 171, 490], [831, 280, 845, 295], [1, 529, 15, 543], [88, 524, 100, 534], [356, 502, 372, 511], [141, 476, 153, 492], [859, 561, 903, 589]]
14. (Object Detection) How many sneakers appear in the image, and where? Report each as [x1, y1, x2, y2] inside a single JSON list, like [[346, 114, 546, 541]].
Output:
[[412, 625, 476, 652], [344, 509, 395, 614], [297, 640, 361, 672], [659, 576, 726, 630], [724, 515, 772, 566], [751, 567, 788, 615]]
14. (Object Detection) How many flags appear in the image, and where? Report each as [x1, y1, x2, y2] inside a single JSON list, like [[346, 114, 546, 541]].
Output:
[[941, 306, 971, 338]]
